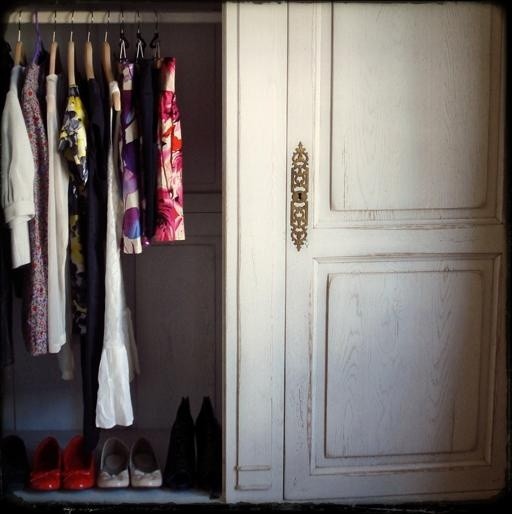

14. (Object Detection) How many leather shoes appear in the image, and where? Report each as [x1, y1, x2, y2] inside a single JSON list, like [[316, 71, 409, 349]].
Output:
[[96, 436, 130, 489], [28, 436, 64, 491], [61, 435, 97, 491], [129, 436, 164, 489]]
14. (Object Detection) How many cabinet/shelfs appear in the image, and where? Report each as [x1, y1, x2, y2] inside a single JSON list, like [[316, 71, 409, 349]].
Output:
[[2, 0, 508, 508]]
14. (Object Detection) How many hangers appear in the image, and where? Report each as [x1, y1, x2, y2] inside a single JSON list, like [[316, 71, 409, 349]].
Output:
[[10, 7, 163, 86]]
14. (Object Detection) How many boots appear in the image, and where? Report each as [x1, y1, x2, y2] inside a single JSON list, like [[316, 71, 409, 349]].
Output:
[[194, 394, 223, 494], [163, 395, 197, 491]]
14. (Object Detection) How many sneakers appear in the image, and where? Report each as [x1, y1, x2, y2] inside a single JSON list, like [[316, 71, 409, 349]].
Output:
[[1, 434, 29, 492]]
